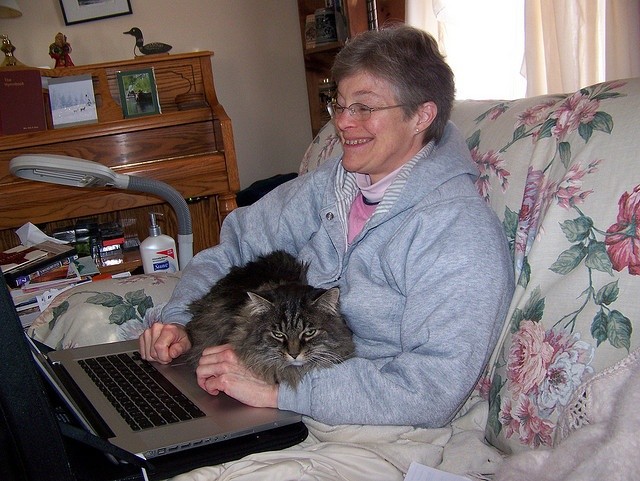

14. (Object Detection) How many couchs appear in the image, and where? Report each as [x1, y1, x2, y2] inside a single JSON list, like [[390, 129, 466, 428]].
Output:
[[23, 64, 639, 481]]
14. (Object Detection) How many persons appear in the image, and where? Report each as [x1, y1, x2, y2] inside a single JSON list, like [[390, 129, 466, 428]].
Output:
[[48, 33, 75, 68], [139, 18, 515, 481]]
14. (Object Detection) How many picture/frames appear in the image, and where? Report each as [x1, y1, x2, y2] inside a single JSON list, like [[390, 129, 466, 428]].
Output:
[[115, 66, 163, 120], [58, 0, 133, 27]]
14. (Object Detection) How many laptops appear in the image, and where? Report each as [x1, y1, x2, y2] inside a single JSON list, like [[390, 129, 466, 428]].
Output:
[[23, 331, 302, 465]]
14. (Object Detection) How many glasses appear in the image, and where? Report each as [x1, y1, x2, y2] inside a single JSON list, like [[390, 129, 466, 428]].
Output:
[[326, 99, 426, 122]]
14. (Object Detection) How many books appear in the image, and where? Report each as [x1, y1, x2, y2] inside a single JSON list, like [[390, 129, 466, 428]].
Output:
[[1, 240, 101, 330]]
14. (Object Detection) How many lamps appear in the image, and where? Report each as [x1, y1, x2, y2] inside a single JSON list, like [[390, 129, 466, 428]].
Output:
[[7, 152, 194, 272], [0, 0, 27, 65]]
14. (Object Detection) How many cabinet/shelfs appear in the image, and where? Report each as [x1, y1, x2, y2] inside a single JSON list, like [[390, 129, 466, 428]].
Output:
[[1, 50, 241, 258], [297, 1, 407, 143]]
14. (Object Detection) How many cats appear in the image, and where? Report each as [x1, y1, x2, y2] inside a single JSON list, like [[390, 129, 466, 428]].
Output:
[[179, 248, 354, 392]]
